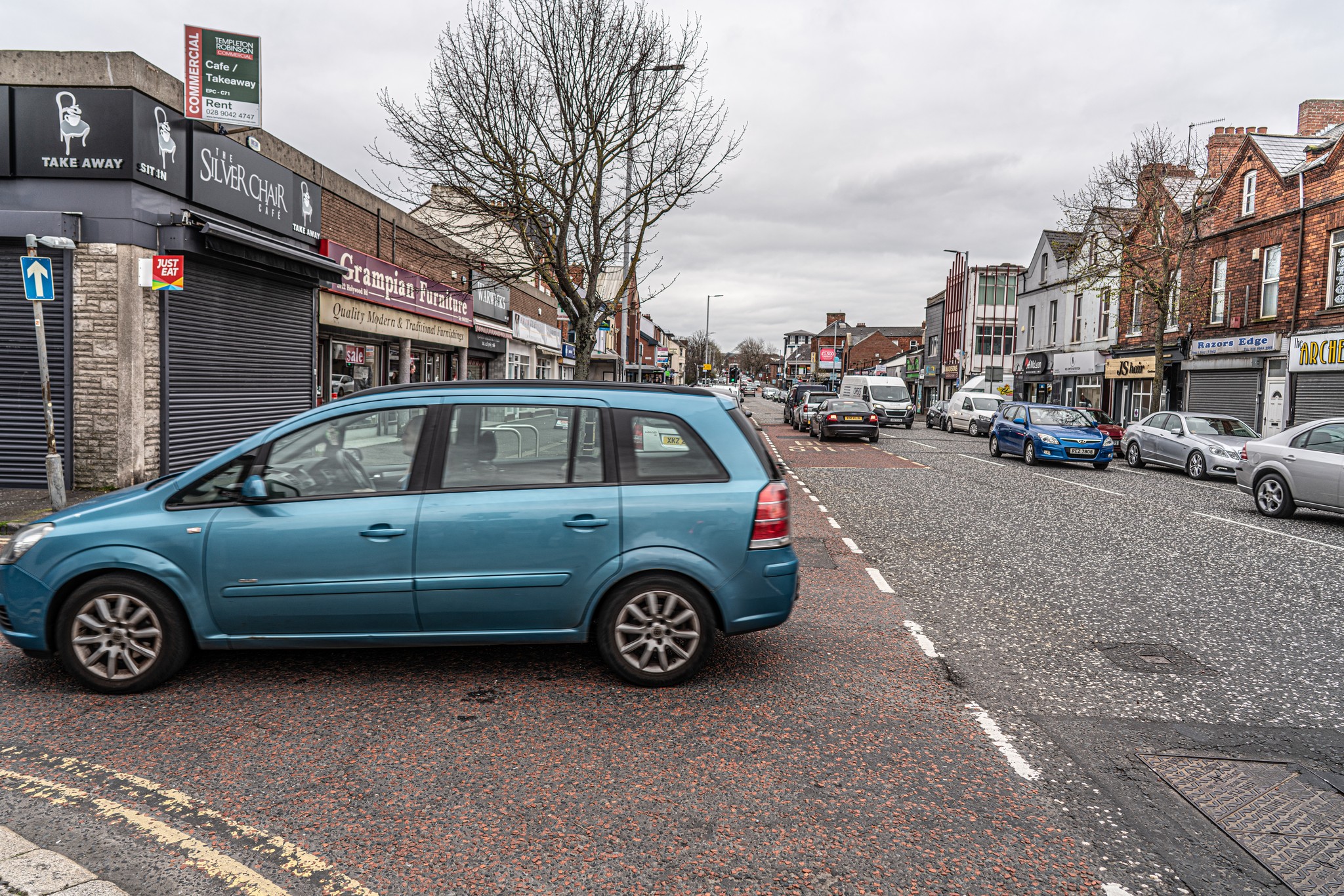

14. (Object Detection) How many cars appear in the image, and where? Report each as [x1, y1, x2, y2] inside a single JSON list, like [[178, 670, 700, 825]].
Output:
[[740, 371, 745, 377], [989, 402, 1125, 470], [741, 375, 751, 383], [810, 398, 879, 443], [926, 399, 951, 431], [1234, 417, 1344, 519], [1120, 411, 1265, 480], [740, 380, 761, 397], [761, 387, 790, 403]]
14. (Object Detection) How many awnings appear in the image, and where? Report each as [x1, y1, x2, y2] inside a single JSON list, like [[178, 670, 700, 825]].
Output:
[[184, 208, 348, 285]]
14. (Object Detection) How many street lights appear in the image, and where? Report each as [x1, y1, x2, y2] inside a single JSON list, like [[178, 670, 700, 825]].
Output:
[[765, 364, 769, 387], [792, 343, 797, 379], [944, 249, 968, 391], [825, 321, 837, 393], [705, 295, 724, 387], [776, 349, 781, 388]]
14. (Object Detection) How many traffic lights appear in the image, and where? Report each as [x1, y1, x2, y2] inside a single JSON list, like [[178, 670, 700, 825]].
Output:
[[729, 367, 737, 383], [737, 370, 740, 380], [750, 372, 752, 374]]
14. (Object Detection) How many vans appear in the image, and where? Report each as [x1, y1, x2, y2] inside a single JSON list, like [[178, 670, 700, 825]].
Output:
[[946, 391, 1006, 437], [840, 376, 915, 429], [959, 372, 1014, 402], [783, 382, 827, 426]]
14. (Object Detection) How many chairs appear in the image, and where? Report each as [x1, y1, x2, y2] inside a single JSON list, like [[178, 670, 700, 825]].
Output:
[[1024, 412, 1038, 422], [446, 431, 497, 485], [1187, 423, 1198, 432], [1306, 432, 1331, 451], [1171, 420, 1180, 429], [1206, 422, 1223, 434], [834, 404, 860, 411], [811, 397, 817, 402], [1155, 420, 1163, 428]]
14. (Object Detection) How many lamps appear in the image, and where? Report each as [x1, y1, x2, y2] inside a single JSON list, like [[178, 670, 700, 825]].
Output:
[[950, 360, 958, 363], [938, 362, 943, 364], [36, 236, 77, 249]]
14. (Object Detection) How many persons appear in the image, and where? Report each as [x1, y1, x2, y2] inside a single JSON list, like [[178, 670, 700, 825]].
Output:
[[760, 377, 762, 381], [365, 371, 373, 389], [872, 387, 879, 395], [661, 382, 667, 384], [648, 373, 663, 383], [390, 371, 398, 385], [1080, 395, 1092, 408], [409, 363, 415, 383], [352, 415, 426, 493], [466, 369, 476, 380]]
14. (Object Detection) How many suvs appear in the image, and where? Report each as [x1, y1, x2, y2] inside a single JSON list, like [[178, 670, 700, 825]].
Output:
[[692, 384, 744, 412], [793, 390, 839, 432], [1134, 406, 1153, 422], [632, 416, 690, 458], [0, 379, 801, 696]]
